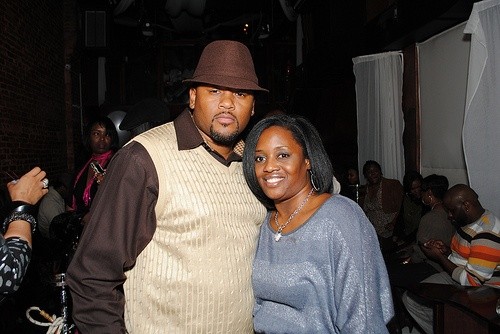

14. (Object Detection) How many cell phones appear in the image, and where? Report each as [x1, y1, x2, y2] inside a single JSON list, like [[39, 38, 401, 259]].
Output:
[[417, 241, 428, 249], [91, 160, 103, 174]]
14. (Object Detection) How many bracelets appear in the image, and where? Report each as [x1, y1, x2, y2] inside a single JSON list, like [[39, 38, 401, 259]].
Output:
[[3, 211, 36, 233]]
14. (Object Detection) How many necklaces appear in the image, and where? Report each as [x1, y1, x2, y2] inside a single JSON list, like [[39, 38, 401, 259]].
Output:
[[273, 187, 315, 242]]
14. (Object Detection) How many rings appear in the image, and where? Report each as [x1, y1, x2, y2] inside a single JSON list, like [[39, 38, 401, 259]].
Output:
[[42, 178, 49, 189]]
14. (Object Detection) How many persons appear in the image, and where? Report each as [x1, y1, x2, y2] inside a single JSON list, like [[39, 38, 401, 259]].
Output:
[[241, 112, 396, 334], [351, 159, 500, 334], [0, 112, 121, 334], [63, 40, 269, 334]]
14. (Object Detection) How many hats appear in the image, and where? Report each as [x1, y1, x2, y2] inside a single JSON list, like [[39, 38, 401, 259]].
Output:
[[181, 40, 269, 91]]
